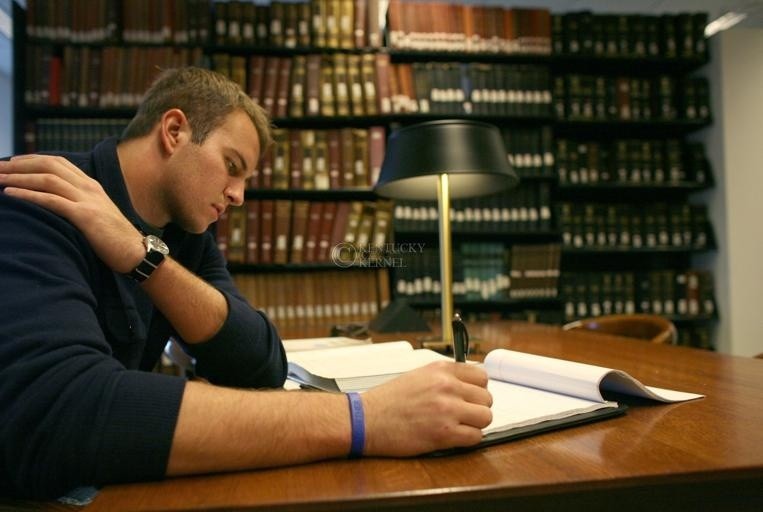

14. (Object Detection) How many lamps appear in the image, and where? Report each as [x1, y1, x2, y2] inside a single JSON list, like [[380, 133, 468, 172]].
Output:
[[372, 119, 520, 355]]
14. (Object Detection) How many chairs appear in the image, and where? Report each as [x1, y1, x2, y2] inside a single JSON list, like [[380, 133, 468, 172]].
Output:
[[562, 314, 678, 347]]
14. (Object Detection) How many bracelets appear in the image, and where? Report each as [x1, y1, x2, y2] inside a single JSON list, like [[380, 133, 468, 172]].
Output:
[[346, 392, 365, 457]]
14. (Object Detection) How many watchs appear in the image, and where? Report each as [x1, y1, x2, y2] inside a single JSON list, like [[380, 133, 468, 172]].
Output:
[[127, 234, 170, 284]]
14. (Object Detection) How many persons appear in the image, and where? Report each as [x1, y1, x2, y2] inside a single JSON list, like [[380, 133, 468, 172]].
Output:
[[0, 67, 492, 498]]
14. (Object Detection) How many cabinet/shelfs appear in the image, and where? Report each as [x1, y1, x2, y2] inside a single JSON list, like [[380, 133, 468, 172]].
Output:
[[0, 0, 719, 350]]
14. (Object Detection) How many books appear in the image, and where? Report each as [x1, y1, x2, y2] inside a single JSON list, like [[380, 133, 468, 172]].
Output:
[[20, 116, 130, 154], [278, 328, 707, 443], [500, 125, 716, 187], [232, 266, 387, 341], [386, 1, 715, 120], [388, 238, 720, 353], [20, 0, 404, 122], [209, 127, 393, 263], [396, 189, 714, 251]]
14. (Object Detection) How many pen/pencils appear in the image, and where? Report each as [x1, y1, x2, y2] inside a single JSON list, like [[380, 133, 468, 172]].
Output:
[[452, 312, 469, 362]]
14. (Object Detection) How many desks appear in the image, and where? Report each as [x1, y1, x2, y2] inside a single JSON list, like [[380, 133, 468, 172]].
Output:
[[43, 320, 762, 511]]
